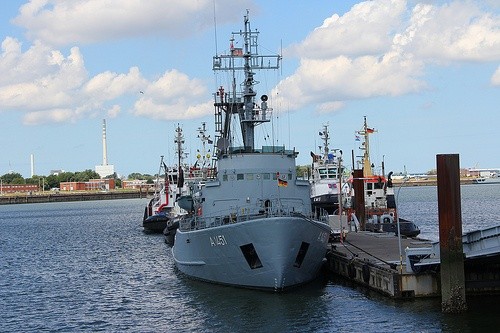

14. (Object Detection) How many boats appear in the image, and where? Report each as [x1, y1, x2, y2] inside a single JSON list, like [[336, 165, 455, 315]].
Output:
[[475, 171, 500, 183], [171, 0, 333, 294], [310, 115, 421, 241], [143, 121, 218, 247]]
